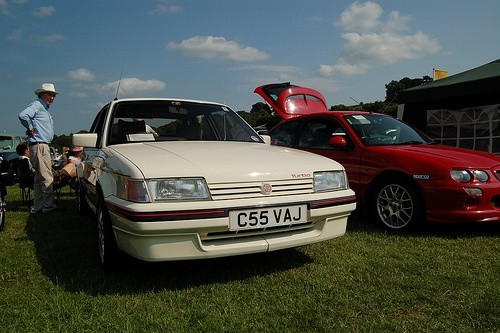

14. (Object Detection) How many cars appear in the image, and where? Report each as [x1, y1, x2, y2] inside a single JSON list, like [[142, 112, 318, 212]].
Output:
[[252, 81, 500, 237], [0, 133, 26, 160], [71, 97, 357, 272]]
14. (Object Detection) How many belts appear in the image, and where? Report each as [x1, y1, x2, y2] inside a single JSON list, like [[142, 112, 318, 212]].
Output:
[[31, 142, 48, 145]]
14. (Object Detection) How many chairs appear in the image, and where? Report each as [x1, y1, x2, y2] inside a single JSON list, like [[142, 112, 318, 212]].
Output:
[[175, 123, 202, 140], [308, 128, 332, 146], [116, 119, 146, 144], [9, 157, 77, 216]]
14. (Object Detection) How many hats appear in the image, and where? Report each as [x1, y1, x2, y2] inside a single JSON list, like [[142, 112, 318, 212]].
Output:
[[35, 83, 61, 95], [72, 146, 84, 151]]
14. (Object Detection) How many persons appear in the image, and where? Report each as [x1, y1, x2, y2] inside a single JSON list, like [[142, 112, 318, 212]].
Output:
[[16, 142, 82, 190], [18, 83, 61, 214]]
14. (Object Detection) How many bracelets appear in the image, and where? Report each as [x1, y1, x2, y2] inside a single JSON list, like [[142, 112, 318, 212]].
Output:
[[30, 129, 33, 132]]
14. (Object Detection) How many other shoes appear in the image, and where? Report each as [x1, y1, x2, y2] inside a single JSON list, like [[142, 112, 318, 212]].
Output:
[[43, 207, 61, 213], [35, 208, 42, 213]]
